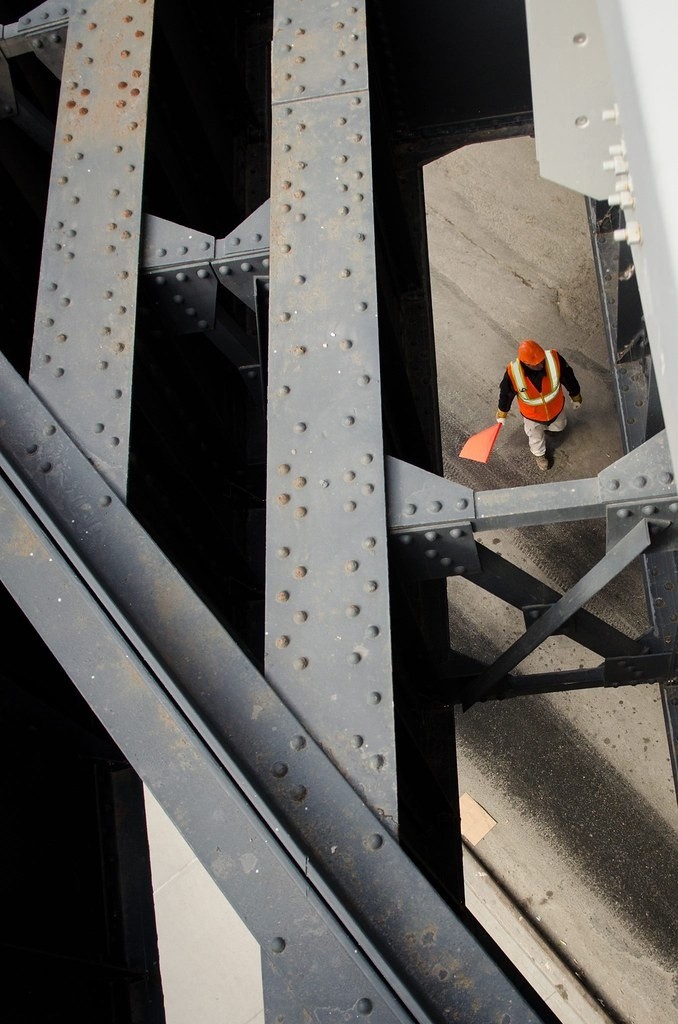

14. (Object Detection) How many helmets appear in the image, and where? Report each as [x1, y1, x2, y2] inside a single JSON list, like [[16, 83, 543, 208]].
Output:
[[518, 339, 545, 365]]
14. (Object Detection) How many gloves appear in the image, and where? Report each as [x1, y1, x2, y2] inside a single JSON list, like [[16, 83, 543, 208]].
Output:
[[571, 393, 583, 410], [496, 408, 508, 425]]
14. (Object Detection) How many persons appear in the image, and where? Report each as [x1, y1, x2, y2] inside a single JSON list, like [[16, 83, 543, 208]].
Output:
[[495, 340, 584, 472]]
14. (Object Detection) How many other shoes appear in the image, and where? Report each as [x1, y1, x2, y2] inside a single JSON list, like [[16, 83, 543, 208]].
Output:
[[535, 455, 549, 471]]
